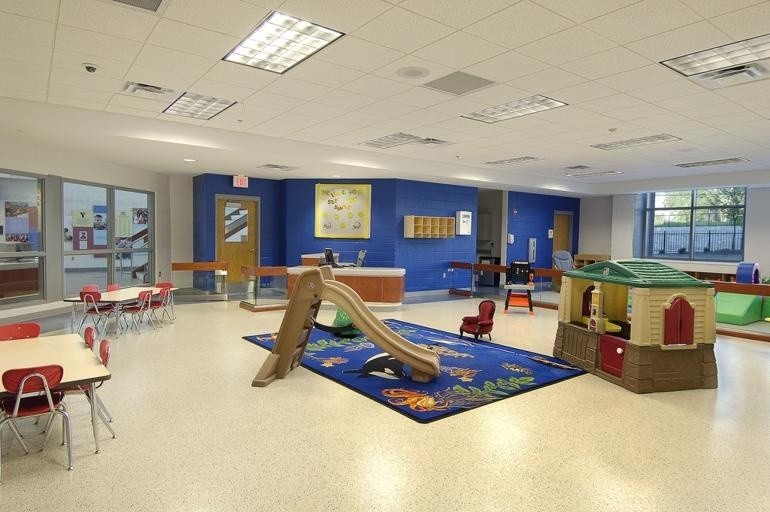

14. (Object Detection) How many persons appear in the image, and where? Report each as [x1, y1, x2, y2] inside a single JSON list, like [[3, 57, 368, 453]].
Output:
[[94, 215, 108, 230]]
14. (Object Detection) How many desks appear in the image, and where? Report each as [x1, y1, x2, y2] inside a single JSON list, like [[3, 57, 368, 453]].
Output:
[[285, 252, 406, 310]]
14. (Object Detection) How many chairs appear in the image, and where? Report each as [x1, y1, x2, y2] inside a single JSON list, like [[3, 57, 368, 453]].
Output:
[[551, 249, 577, 294], [356, 249, 368, 266], [457, 299, 496, 342], [502, 259, 536, 316], [1, 282, 180, 471]]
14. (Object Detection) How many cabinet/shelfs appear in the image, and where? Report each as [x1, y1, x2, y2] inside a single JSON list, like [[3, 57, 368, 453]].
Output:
[[573, 254, 610, 270], [403, 215, 456, 240]]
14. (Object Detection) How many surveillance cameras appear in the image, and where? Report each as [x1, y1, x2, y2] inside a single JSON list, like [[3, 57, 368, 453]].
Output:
[[82, 63, 98, 73]]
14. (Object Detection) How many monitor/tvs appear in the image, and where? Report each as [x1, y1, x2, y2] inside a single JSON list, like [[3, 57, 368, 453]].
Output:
[[324, 248, 335, 267]]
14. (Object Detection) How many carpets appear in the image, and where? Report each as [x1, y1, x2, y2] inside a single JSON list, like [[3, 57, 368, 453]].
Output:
[[242, 318, 587, 424]]
